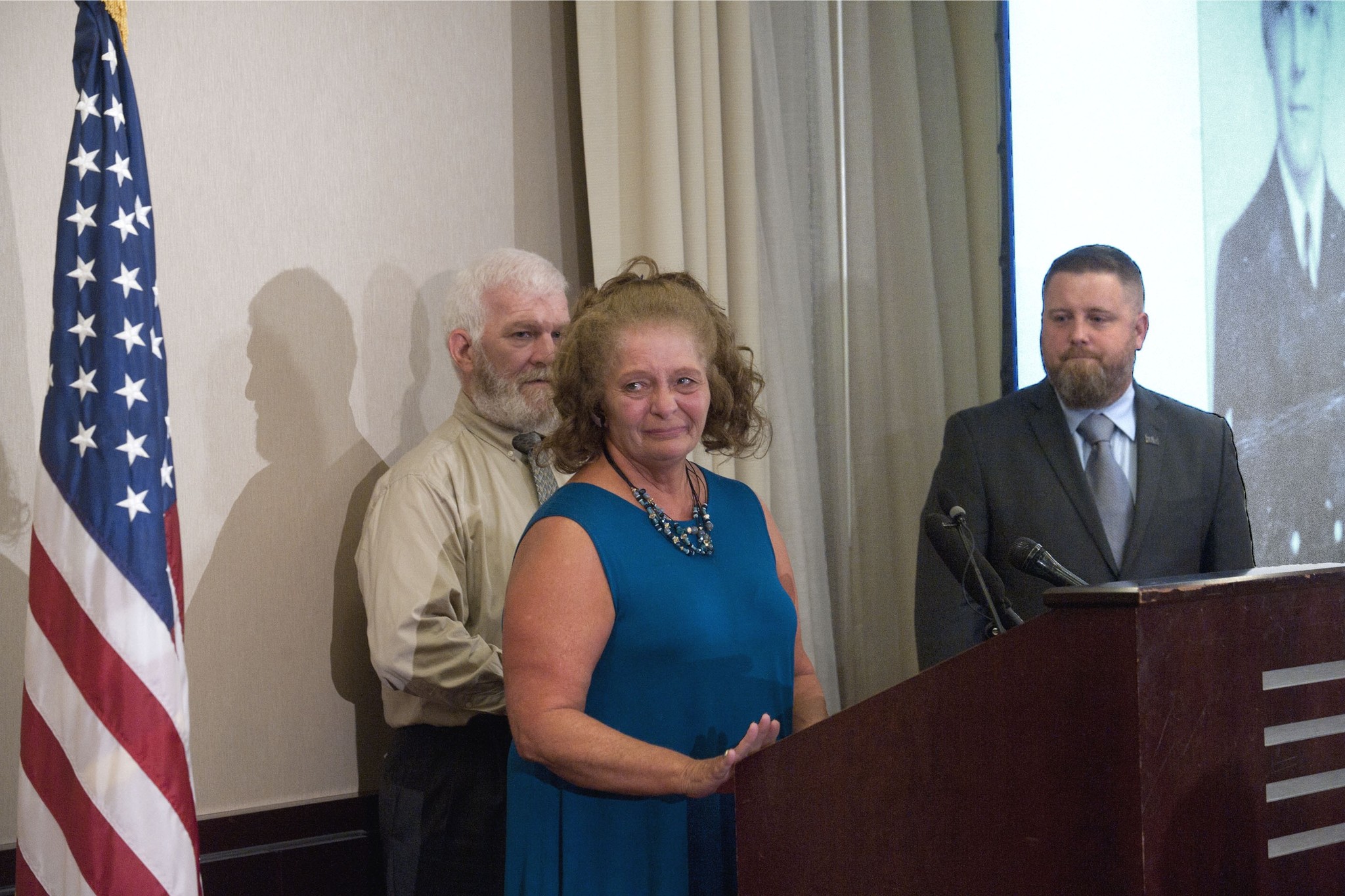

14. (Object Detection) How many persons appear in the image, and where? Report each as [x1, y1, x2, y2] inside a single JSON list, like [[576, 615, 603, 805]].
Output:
[[502, 256, 830, 896], [911, 243, 1258, 676], [356, 249, 601, 896], [1209, 0, 1345, 565]]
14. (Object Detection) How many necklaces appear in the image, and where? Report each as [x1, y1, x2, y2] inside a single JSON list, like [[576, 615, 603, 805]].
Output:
[[599, 437, 714, 557]]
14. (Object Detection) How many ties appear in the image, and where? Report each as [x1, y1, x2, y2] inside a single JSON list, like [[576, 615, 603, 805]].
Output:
[[512, 431, 558, 507], [1303, 211, 1314, 291]]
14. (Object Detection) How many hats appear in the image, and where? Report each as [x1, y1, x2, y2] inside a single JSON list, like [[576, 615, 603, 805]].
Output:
[[1077, 414, 1134, 573]]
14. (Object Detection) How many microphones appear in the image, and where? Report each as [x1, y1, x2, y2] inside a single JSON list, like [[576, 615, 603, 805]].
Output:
[[924, 514, 1024, 631], [1008, 537, 1090, 587], [951, 504, 1007, 632]]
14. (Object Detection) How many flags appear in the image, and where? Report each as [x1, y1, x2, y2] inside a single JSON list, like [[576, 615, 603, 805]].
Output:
[[14, 0, 206, 896]]
